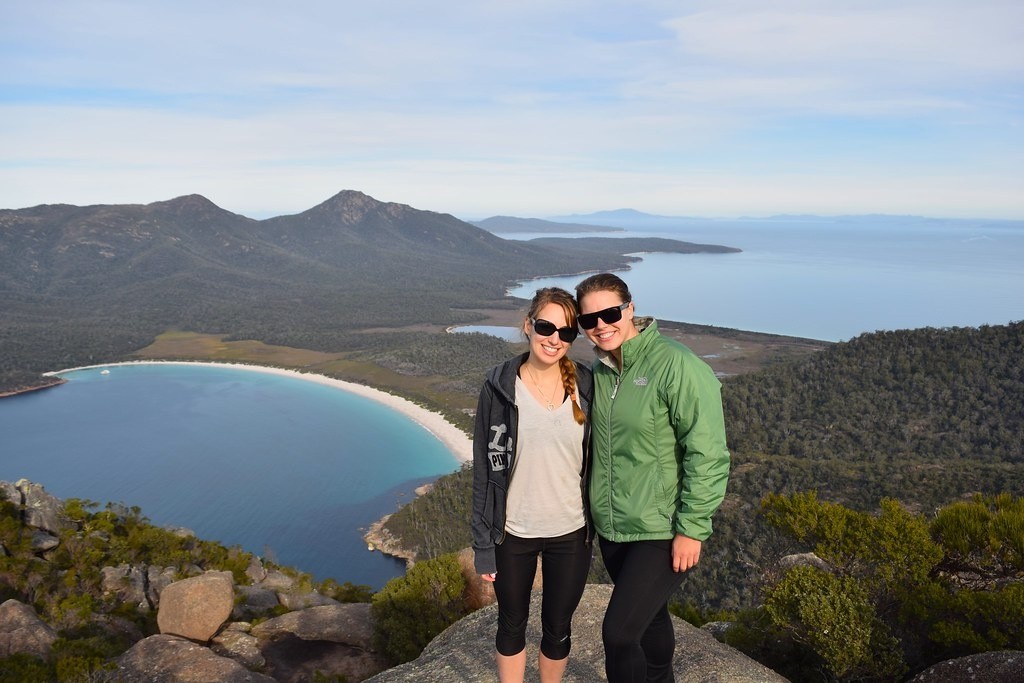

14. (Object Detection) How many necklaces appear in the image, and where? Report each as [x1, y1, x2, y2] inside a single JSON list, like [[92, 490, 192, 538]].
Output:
[[525, 355, 564, 411]]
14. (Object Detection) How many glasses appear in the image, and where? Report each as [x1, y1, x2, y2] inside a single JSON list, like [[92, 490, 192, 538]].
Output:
[[531, 318, 578, 343], [578, 302, 629, 330]]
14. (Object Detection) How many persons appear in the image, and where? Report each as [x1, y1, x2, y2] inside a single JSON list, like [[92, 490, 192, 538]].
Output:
[[472, 285, 597, 683], [573, 273, 731, 683]]
